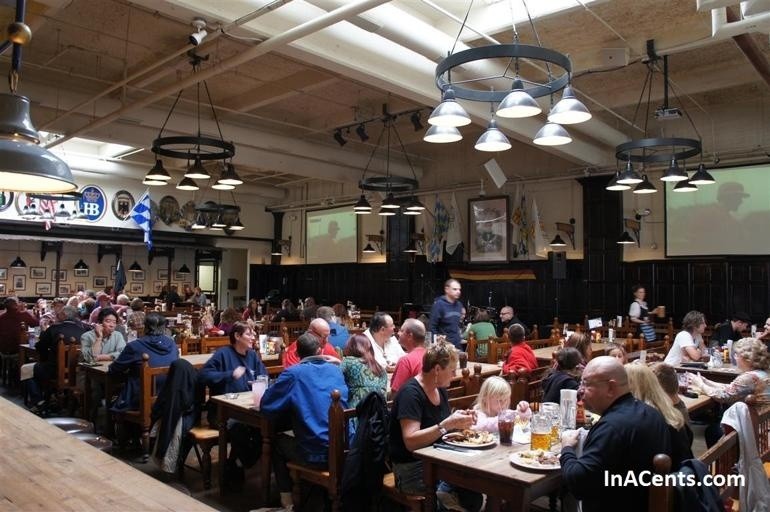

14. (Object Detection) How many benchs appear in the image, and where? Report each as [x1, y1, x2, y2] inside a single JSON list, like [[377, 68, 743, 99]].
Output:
[[489, 330, 556, 366], [519, 380, 547, 408], [69, 333, 188, 417], [180, 359, 284, 493], [468, 325, 537, 362], [57, 332, 70, 391], [286, 368, 470, 512], [381, 370, 517, 512], [474, 366, 501, 392], [107, 344, 284, 463], [555, 308, 744, 352], [647, 393, 770, 512], [242, 304, 404, 342], [200, 336, 232, 353]]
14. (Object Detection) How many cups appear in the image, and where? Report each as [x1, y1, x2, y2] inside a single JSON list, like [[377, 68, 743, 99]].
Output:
[[266, 342, 275, 355], [459, 352, 468, 369], [543, 402, 559, 444], [702, 348, 710, 363], [259, 334, 266, 353], [561, 389, 576, 431], [252, 380, 264, 408], [257, 375, 270, 392], [657, 306, 665, 319], [677, 374, 689, 396], [498, 411, 515, 446], [530, 420, 551, 453]]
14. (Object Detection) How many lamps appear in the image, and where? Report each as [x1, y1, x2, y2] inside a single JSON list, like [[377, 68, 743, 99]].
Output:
[[190, 18, 208, 46], [410, 111, 424, 131], [0, 0, 78, 193], [605, 55, 716, 195], [271, 239, 292, 256], [10, 240, 27, 269], [21, 192, 82, 217], [334, 129, 347, 146], [423, 0, 592, 153], [362, 234, 384, 255], [616, 218, 641, 248], [177, 246, 191, 275], [403, 233, 424, 253], [128, 247, 142, 273], [357, 123, 370, 141], [549, 222, 575, 249], [142, 56, 244, 190], [351, 114, 425, 216], [190, 161, 246, 231], [74, 245, 89, 270]]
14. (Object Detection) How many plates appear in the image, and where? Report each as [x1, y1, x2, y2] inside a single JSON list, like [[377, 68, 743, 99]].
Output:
[[509, 451, 561, 469], [442, 431, 497, 448]]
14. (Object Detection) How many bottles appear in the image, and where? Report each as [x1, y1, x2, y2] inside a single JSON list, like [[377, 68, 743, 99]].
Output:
[[723, 348, 729, 362]]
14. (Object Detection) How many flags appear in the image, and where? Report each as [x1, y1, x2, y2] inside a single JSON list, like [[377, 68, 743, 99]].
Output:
[[446, 192, 464, 254], [431, 193, 449, 263], [518, 182, 530, 257], [510, 184, 523, 251], [130, 189, 153, 251], [531, 196, 549, 258], [40, 193, 57, 230], [422, 203, 435, 255]]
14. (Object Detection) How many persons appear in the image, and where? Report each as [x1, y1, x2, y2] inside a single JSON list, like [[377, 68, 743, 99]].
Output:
[[241, 298, 263, 321], [458, 308, 468, 331], [541, 348, 580, 404], [184, 287, 194, 302], [390, 318, 426, 402], [629, 286, 659, 343], [498, 306, 530, 341], [475, 207, 503, 253], [218, 308, 245, 336], [662, 311, 710, 369], [552, 331, 592, 378], [500, 324, 540, 380], [462, 309, 501, 357], [195, 321, 268, 493], [605, 342, 628, 366], [34, 286, 130, 329], [684, 337, 770, 449], [76, 308, 127, 418], [281, 318, 341, 370], [559, 355, 667, 511], [387, 337, 487, 512], [31, 306, 94, 418], [0, 297, 41, 355], [127, 298, 147, 329], [316, 306, 349, 350], [336, 334, 388, 431], [108, 313, 180, 446], [259, 334, 356, 512], [308, 220, 341, 258], [167, 286, 183, 306], [271, 298, 300, 323], [623, 360, 694, 476], [652, 363, 690, 431], [468, 376, 533, 432], [158, 286, 168, 300], [333, 304, 354, 330], [709, 311, 752, 348], [192, 287, 206, 306], [757, 317, 770, 353], [362, 312, 408, 372], [429, 279, 462, 355], [300, 297, 319, 322]]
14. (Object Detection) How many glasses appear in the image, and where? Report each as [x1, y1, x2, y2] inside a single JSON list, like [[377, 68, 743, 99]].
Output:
[[501, 313, 508, 315], [398, 328, 406, 336], [582, 379, 606, 388], [314, 332, 326, 340]]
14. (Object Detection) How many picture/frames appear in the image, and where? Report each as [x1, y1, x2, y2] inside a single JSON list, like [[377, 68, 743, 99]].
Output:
[[174, 271, 185, 281], [74, 270, 89, 277], [153, 281, 163, 293], [51, 269, 68, 281], [59, 284, 71, 293], [75, 282, 85, 291], [467, 195, 512, 266], [130, 283, 143, 293], [13, 275, 26, 290], [93, 276, 107, 288], [35, 282, 52, 295], [171, 284, 177, 287], [0, 283, 6, 295], [111, 266, 116, 280], [0, 268, 8, 280], [158, 268, 168, 280], [30, 266, 46, 279], [132, 270, 146, 280], [182, 282, 191, 293]]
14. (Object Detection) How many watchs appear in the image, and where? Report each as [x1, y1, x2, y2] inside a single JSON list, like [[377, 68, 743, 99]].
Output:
[[437, 423, 447, 435]]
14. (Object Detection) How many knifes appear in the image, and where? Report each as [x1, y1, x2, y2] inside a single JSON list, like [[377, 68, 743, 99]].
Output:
[[434, 443, 467, 452]]
